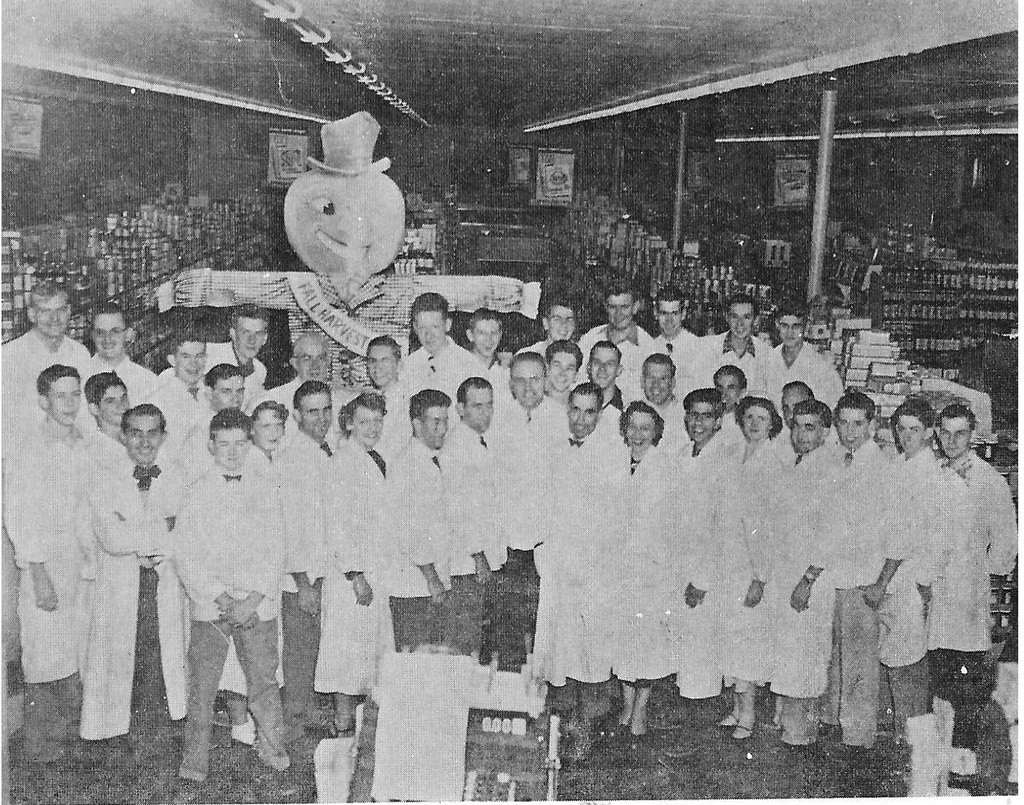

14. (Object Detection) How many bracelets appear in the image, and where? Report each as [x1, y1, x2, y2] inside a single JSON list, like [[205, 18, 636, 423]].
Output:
[[804, 575, 816, 585]]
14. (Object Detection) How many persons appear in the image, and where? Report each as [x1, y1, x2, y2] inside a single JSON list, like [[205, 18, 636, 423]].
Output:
[[1, 283, 1018, 804]]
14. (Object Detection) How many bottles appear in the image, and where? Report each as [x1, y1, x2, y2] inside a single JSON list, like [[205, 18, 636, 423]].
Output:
[[382, 205, 443, 278], [2, 199, 283, 353], [822, 227, 1018, 634], [572, 193, 782, 331]]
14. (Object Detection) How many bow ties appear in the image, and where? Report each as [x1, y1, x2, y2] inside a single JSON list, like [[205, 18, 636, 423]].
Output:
[[133, 465, 161, 490], [568, 438, 585, 448], [223, 474, 241, 482]]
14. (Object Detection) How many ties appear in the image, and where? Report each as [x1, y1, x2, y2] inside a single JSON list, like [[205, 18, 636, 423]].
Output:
[[431, 456, 440, 469], [320, 441, 333, 458], [844, 452, 854, 466], [480, 436, 488, 448], [188, 387, 199, 402], [666, 343, 673, 354], [794, 454, 803, 465]]
